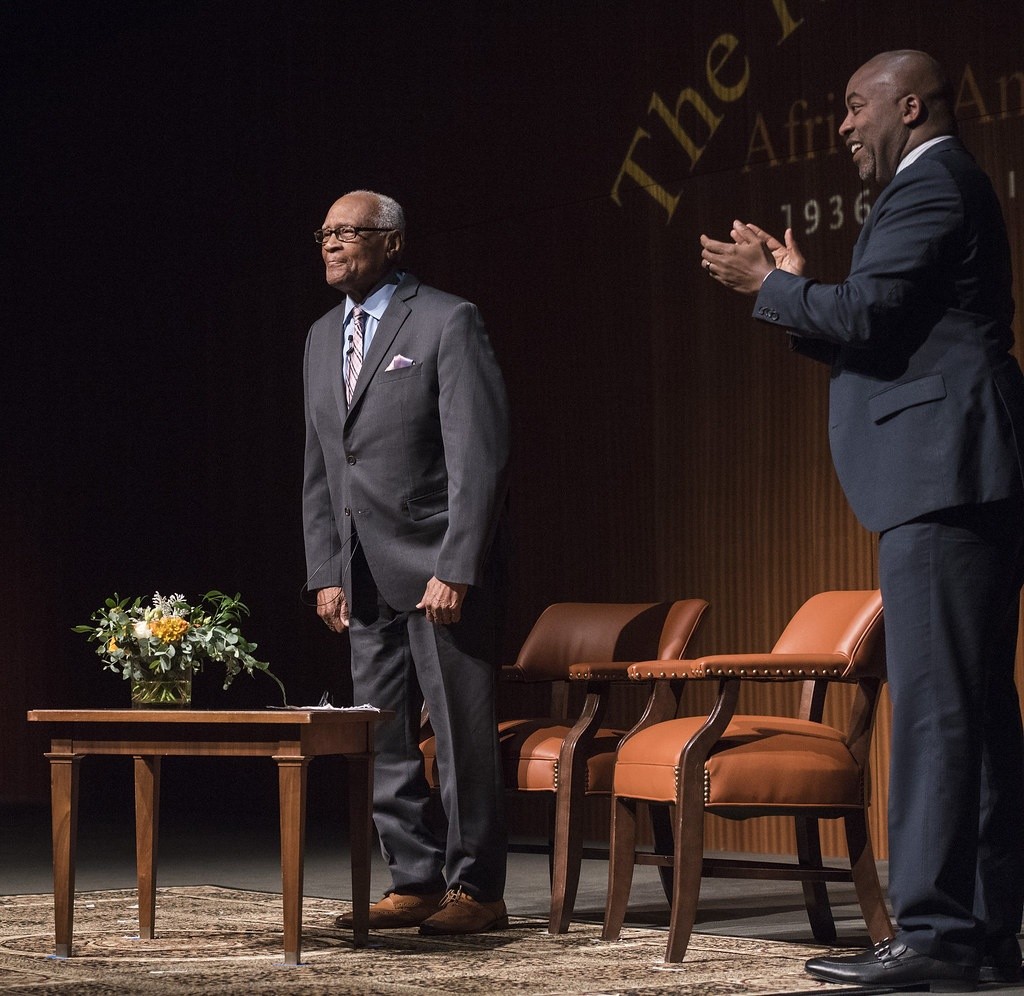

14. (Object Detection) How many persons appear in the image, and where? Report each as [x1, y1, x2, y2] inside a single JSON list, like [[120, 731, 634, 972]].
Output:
[[702, 50, 1024, 996], [304, 191, 509, 935]]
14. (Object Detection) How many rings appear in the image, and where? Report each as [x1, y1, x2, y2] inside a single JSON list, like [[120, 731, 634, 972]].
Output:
[[706, 261, 711, 271]]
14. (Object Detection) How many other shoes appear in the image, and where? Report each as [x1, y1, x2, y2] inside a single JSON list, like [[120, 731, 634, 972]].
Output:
[[417, 889, 510, 936], [333, 887, 450, 930]]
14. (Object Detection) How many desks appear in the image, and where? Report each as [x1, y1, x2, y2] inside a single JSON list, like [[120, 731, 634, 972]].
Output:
[[28, 707, 398, 964]]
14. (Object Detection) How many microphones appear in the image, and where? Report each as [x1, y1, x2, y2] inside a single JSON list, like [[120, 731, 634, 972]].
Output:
[[348, 336, 353, 341]]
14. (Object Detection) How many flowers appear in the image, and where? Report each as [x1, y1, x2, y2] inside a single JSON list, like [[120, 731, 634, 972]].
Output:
[[73, 591, 271, 691]]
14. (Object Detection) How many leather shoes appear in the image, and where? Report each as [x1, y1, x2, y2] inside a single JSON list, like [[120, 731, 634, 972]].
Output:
[[803, 931, 984, 995], [977, 960, 1022, 989]]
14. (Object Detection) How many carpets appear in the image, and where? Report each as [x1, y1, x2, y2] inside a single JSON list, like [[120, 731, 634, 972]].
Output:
[[1, 885, 933, 996]]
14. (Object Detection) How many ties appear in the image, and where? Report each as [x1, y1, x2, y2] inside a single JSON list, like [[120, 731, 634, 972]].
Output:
[[343, 306, 368, 414]]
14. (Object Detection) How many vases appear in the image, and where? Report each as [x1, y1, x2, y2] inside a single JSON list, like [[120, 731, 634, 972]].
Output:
[[132, 661, 193, 709]]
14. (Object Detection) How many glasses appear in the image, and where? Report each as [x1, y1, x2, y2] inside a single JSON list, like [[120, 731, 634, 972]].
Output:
[[313, 226, 393, 243]]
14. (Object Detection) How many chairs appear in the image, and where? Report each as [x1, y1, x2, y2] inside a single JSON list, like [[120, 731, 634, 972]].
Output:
[[416, 598, 712, 933], [603, 588, 897, 964]]
